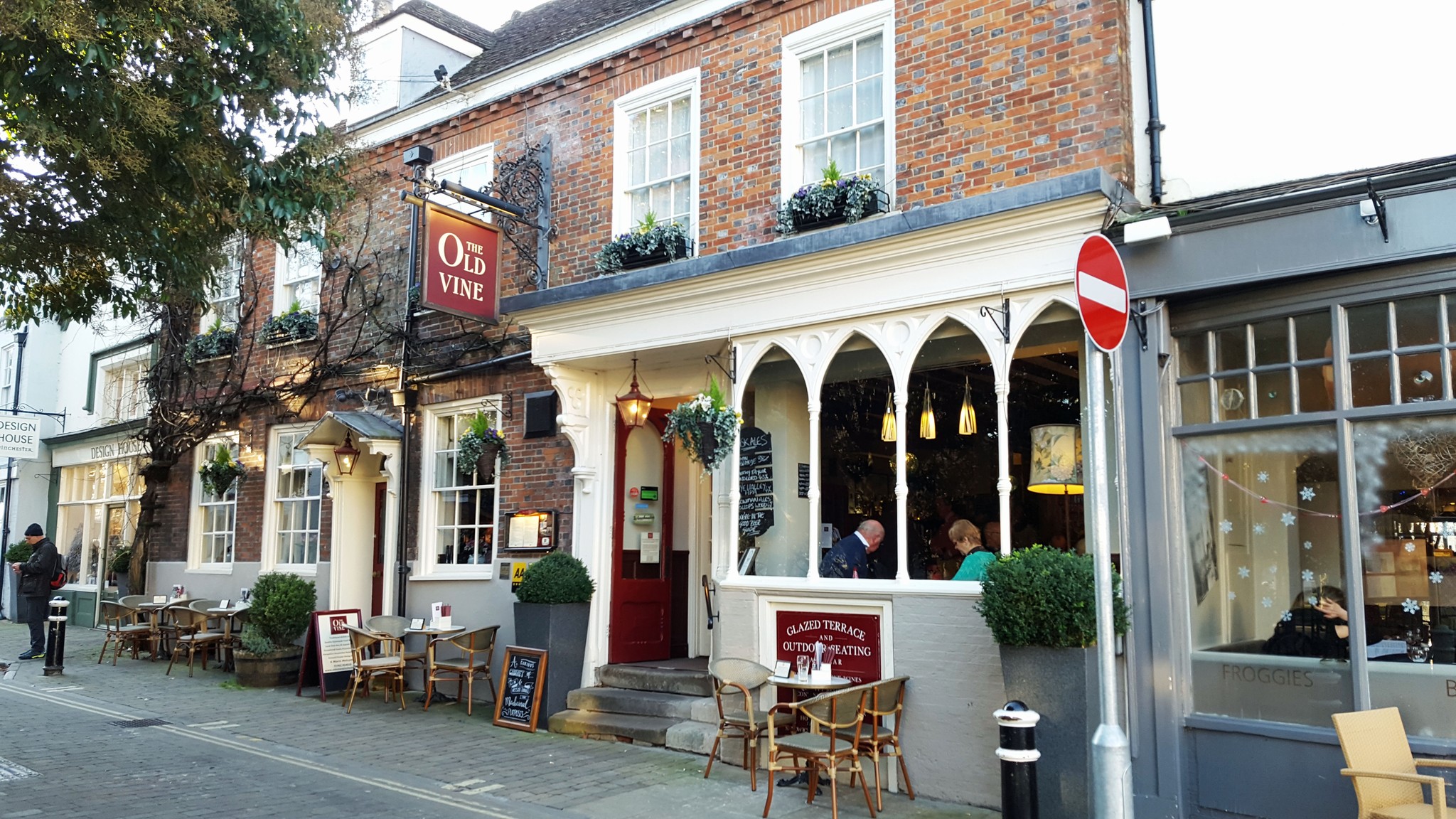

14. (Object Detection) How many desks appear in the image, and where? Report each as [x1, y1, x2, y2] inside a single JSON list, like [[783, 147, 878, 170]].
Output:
[[403, 623, 467, 711], [764, 671, 856, 795]]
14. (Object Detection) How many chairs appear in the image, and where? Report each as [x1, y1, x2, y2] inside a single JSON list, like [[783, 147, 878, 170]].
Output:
[[702, 669, 803, 790], [761, 685, 877, 818], [101, 594, 255, 680], [424, 623, 503, 716], [808, 673, 916, 815], [1331, 704, 1456, 819], [340, 623, 407, 713], [362, 614, 431, 704]]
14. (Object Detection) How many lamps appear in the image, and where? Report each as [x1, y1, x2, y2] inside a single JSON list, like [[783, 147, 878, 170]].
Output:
[[883, 383, 899, 442], [918, 379, 939, 441], [614, 354, 656, 429], [333, 425, 362, 479], [958, 373, 978, 439], [1026, 423, 1085, 553]]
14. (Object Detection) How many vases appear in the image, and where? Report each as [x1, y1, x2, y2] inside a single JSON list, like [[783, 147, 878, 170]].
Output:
[[476, 442, 499, 483], [693, 423, 719, 469], [207, 470, 239, 499]]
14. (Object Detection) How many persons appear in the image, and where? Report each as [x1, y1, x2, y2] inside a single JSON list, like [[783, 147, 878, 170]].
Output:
[[983, 520, 1000, 550], [817, 519, 886, 578], [1274, 585, 1349, 661], [1262, 589, 1318, 655], [948, 519, 998, 582], [12, 523, 57, 661]]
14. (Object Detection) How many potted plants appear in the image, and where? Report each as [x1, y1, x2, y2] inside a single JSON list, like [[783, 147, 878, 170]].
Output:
[[977, 542, 1137, 817], [259, 301, 317, 350], [778, 154, 882, 238], [183, 317, 239, 364], [594, 212, 690, 280], [231, 572, 318, 686], [514, 548, 596, 733]]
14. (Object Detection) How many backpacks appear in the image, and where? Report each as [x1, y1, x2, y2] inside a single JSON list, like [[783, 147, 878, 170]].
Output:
[[41, 541, 68, 590]]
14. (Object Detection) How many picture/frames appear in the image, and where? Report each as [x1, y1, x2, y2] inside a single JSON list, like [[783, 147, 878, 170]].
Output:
[[491, 644, 549, 733]]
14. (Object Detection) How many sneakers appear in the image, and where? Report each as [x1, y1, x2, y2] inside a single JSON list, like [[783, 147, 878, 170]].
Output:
[[18, 646, 46, 659]]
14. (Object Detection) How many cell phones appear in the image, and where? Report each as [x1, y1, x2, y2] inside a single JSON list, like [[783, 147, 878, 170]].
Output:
[[7, 562, 12, 566]]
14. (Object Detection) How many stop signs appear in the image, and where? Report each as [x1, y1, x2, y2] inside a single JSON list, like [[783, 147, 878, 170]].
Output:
[[1073, 228, 1132, 356]]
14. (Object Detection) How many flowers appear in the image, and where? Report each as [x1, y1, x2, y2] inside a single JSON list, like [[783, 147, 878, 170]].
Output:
[[454, 407, 507, 483], [199, 440, 248, 499], [661, 373, 744, 489]]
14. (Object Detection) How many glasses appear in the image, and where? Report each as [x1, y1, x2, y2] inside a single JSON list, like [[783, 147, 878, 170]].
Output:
[[24, 536, 33, 541]]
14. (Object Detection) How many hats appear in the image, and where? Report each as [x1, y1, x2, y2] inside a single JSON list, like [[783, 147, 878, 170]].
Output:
[[24, 523, 43, 536]]
